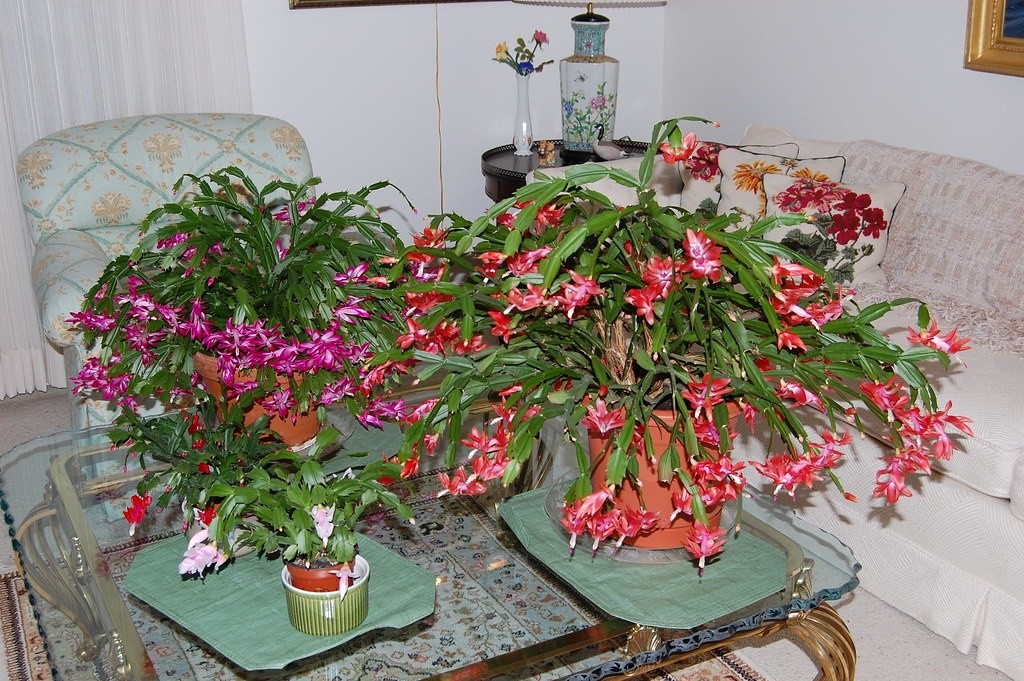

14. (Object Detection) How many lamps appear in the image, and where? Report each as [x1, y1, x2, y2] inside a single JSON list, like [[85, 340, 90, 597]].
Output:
[[514, 1, 667, 149]]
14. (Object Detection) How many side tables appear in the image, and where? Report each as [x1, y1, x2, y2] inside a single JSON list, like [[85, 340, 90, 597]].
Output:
[[480, 139, 665, 235]]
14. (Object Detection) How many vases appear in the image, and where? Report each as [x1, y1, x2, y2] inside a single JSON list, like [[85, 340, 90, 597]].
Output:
[[514, 73, 535, 157], [191, 348, 321, 456], [284, 538, 358, 593], [586, 398, 743, 550]]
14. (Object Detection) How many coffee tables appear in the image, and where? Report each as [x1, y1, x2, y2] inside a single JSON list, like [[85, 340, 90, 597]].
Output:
[[0, 384, 861, 681]]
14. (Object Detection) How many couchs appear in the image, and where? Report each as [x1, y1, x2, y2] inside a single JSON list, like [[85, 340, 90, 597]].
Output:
[[17, 112, 316, 431], [521, 124, 1024, 681]]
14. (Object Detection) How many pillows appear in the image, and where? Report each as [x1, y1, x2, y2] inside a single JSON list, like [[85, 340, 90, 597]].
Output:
[[677, 140, 800, 213], [758, 172, 907, 282], [715, 144, 845, 232]]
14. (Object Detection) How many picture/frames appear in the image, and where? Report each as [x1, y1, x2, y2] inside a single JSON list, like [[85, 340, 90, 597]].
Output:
[[962, 0, 1024, 77]]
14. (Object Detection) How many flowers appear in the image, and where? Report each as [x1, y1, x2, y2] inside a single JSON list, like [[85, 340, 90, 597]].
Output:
[[65, 112, 974, 584], [490, 29, 555, 78], [775, 179, 852, 215], [683, 144, 727, 180], [831, 192, 889, 245], [590, 81, 615, 140], [732, 160, 829, 206]]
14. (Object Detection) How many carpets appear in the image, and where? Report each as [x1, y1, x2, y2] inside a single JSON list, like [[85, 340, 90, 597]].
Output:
[[0, 454, 770, 681]]
